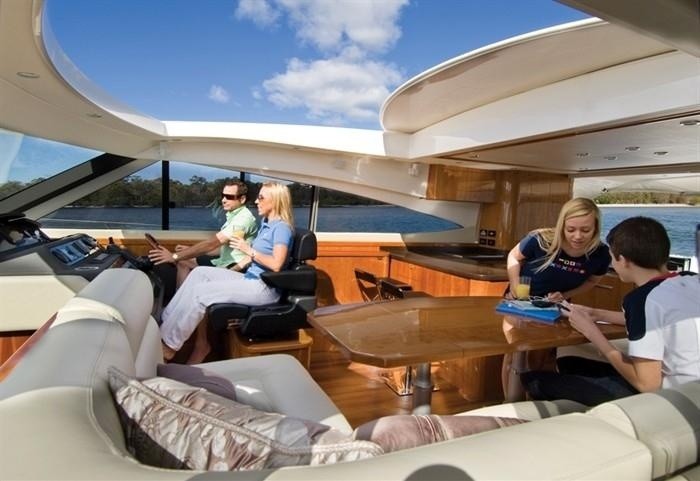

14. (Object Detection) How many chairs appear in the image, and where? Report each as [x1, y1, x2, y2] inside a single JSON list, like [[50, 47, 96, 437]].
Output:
[[198, 228, 318, 361], [354, 268, 413, 305], [380, 280, 459, 397]]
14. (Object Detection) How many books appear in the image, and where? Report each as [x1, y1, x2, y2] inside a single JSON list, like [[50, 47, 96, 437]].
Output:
[[495, 302, 561, 323]]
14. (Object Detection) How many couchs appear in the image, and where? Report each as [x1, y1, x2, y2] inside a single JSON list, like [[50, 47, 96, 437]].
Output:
[[0, 267, 699, 481]]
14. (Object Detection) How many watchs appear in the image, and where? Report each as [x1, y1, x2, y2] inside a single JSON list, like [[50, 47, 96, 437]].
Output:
[[250, 250, 256, 262], [172, 253, 179, 264]]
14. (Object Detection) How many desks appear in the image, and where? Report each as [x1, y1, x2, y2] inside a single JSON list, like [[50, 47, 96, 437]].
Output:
[[307, 293, 627, 416]]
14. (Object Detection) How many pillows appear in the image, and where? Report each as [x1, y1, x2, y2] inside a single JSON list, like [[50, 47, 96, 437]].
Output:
[[107, 365, 383, 473], [155, 361, 270, 412], [351, 412, 532, 454]]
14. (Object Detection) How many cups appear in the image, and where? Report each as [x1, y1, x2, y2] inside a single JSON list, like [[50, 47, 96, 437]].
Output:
[[514, 277, 531, 299], [233, 226, 245, 238]]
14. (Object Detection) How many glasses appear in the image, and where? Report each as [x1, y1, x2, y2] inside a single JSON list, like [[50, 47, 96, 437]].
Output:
[[221, 193, 235, 200]]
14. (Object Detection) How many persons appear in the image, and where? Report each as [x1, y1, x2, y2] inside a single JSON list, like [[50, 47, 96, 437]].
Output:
[[159, 180, 297, 361], [554, 214, 700, 407], [147, 181, 259, 364], [500, 196, 612, 400]]
